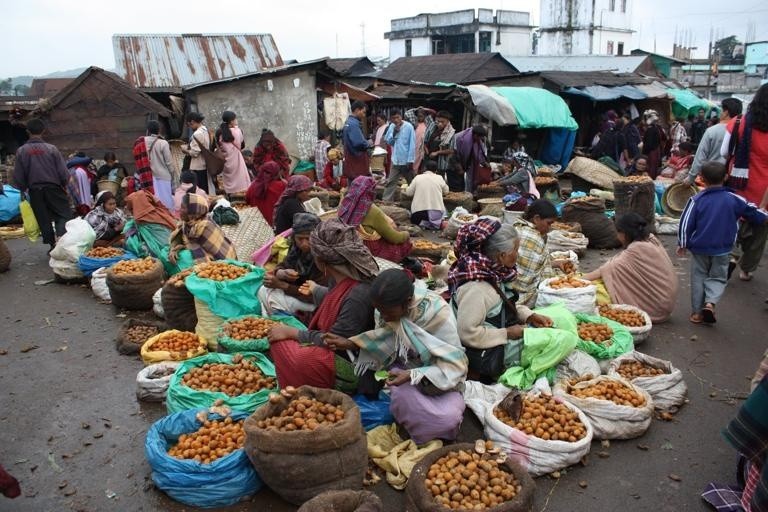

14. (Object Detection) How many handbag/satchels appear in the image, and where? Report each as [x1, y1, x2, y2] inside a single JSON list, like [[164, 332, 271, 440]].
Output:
[[473, 140, 492, 184]]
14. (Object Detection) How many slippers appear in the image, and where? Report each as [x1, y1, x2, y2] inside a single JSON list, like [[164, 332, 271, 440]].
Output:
[[690, 312, 704, 323], [728, 262, 736, 279], [703, 307, 716, 324], [740, 272, 753, 281]]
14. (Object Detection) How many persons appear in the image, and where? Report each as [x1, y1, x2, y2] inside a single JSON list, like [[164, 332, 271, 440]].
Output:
[[400, 161, 449, 229], [675, 161, 766, 323], [684, 113, 701, 140], [605, 108, 619, 122], [695, 107, 712, 128], [168, 192, 238, 265], [320, 268, 468, 448], [446, 218, 577, 389], [314, 132, 332, 183], [505, 137, 526, 156], [403, 108, 433, 175], [135, 120, 175, 211], [97, 152, 128, 184], [477, 151, 540, 212], [581, 209, 677, 323], [338, 176, 413, 264], [268, 217, 379, 396], [512, 199, 558, 309], [120, 176, 175, 230], [245, 162, 288, 227], [272, 175, 313, 236], [14, 118, 70, 257], [370, 114, 391, 167], [214, 111, 246, 192], [627, 155, 651, 177], [589, 120, 621, 161], [682, 98, 743, 191], [68, 152, 96, 208], [491, 157, 515, 182], [317, 149, 349, 191], [85, 191, 128, 242], [666, 113, 687, 161], [448, 124, 492, 192], [421, 110, 455, 177], [263, 213, 329, 304], [181, 111, 210, 196], [343, 100, 375, 186], [383, 110, 416, 204], [252, 128, 293, 178], [719, 83, 768, 281], [616, 114, 643, 157], [636, 110, 669, 180]]
